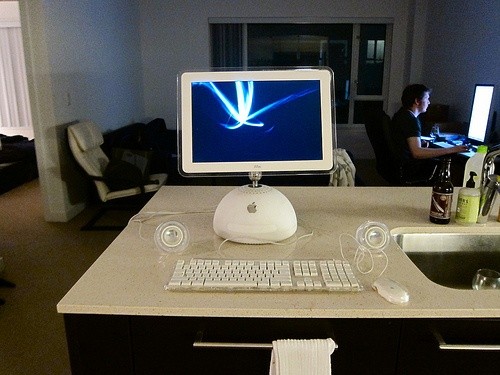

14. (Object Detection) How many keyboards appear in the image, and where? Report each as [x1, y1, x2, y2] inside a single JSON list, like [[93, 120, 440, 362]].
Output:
[[434, 141, 456, 149], [168, 257, 364, 293]]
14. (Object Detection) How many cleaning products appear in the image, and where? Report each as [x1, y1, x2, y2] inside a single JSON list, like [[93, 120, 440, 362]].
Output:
[[454, 170, 481, 227], [428, 154, 453, 226], [462, 145, 496, 188]]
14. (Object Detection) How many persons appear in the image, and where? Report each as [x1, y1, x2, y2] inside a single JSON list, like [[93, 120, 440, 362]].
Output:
[[391, 84, 470, 187]]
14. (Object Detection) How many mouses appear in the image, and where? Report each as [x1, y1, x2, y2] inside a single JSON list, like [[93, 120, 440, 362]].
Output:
[[373, 278, 410, 304]]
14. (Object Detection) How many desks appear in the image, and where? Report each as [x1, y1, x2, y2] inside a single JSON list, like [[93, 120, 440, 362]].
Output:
[[434, 137, 500, 168]]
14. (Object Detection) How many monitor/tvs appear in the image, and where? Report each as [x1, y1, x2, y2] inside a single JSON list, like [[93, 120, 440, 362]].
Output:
[[468, 84, 497, 148], [174, 66, 338, 245]]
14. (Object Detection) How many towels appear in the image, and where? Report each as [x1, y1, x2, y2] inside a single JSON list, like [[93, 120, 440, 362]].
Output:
[[268, 337, 337, 375], [328, 146, 357, 187]]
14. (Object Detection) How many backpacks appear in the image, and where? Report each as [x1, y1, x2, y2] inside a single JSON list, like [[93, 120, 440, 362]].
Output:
[[102, 147, 153, 188]]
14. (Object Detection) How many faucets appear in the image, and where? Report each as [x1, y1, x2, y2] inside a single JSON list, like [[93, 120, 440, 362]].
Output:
[[476, 143, 500, 225]]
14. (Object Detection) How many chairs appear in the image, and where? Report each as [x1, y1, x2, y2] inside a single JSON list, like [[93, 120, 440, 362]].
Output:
[[68, 120, 165, 231], [365, 108, 444, 187]]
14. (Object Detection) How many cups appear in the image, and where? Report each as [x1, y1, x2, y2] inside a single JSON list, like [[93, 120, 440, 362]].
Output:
[[430, 125, 439, 137], [471, 268, 500, 290]]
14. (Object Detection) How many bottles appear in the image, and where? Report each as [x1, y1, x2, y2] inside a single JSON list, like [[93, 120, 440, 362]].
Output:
[[462, 145, 494, 189], [429, 156, 454, 224]]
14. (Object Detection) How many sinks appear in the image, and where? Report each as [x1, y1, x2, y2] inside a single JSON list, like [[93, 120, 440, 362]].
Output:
[[389, 226, 500, 291]]
